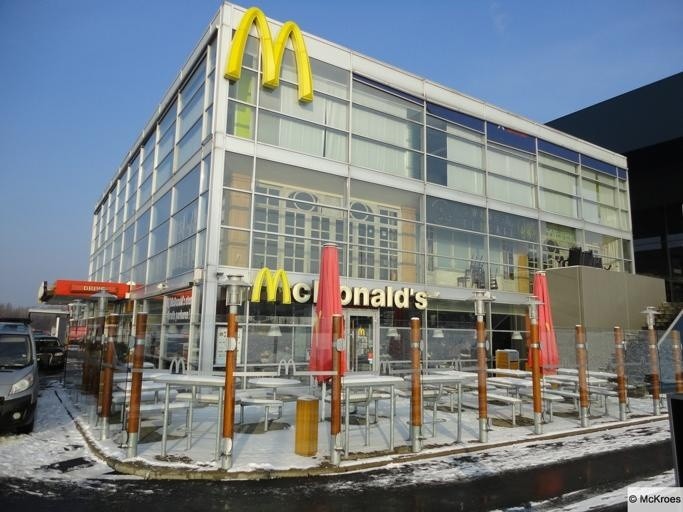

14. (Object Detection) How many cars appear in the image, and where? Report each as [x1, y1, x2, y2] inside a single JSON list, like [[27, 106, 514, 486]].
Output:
[[33, 335, 65, 373]]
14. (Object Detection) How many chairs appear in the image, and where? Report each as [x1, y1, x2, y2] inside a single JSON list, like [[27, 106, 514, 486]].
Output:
[[453, 254, 497, 290]]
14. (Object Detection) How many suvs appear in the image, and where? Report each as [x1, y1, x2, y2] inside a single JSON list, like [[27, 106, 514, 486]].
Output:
[[0, 316, 38, 435]]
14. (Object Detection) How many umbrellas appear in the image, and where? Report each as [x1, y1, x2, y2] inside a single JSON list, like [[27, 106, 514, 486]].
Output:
[[309, 240, 347, 424], [528, 269, 561, 421]]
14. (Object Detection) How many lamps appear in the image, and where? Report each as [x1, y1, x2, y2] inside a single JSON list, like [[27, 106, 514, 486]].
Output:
[[510, 307, 524, 340], [430, 302, 445, 339], [265, 299, 282, 337], [386, 307, 398, 337]]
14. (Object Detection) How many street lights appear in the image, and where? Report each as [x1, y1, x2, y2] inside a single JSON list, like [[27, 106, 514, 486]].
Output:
[[519, 295, 545, 435], [639, 306, 663, 417], [464, 289, 495, 443], [69, 299, 88, 320], [217, 272, 252, 469], [90, 287, 113, 316]]
